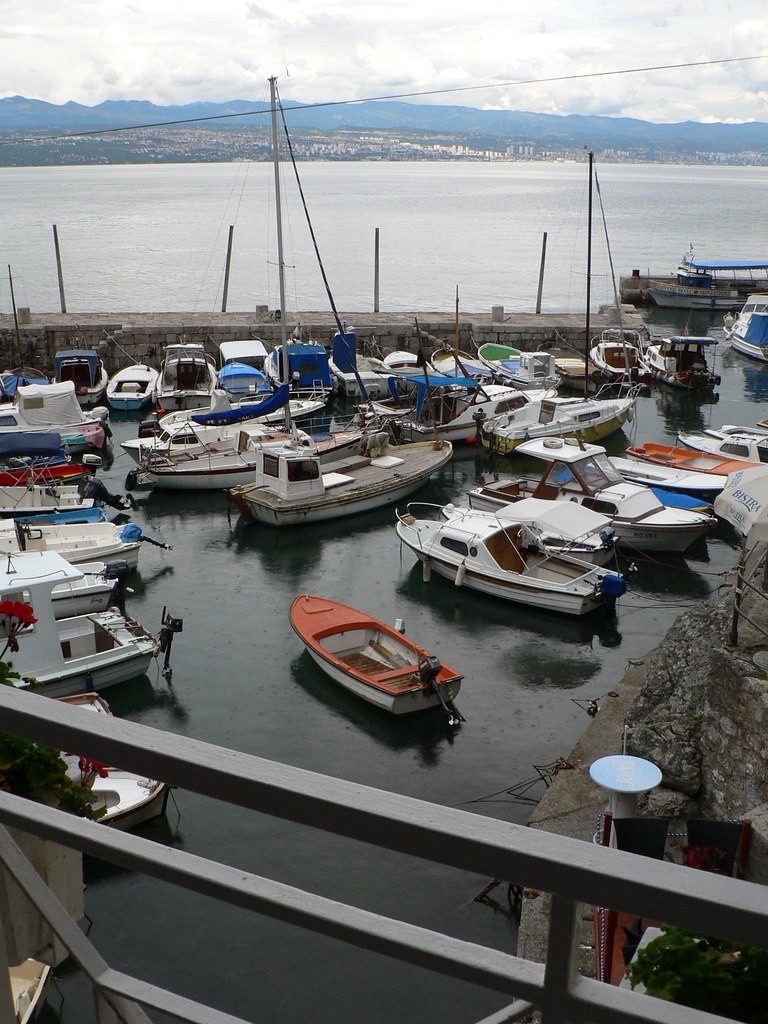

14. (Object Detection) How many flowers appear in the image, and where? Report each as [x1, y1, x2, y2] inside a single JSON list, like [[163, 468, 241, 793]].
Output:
[[0, 598, 110, 823]]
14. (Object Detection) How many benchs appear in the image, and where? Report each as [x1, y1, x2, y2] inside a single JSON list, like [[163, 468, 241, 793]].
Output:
[[482, 478, 520, 496], [666, 450, 701, 465], [370, 661, 418, 681]]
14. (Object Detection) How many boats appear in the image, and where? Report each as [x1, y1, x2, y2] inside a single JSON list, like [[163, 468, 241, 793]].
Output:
[[156, 334, 219, 410], [325, 322, 723, 401], [721, 294, 768, 363], [125, 414, 455, 526], [287, 593, 466, 718], [391, 417, 768, 623], [0, 349, 114, 467], [645, 240, 768, 310], [217, 340, 273, 403], [119, 387, 329, 469], [393, 372, 636, 457], [106, 362, 159, 411], [264, 321, 334, 400], [0, 430, 176, 570], [50, 691, 172, 832], [0, 549, 185, 699]]
